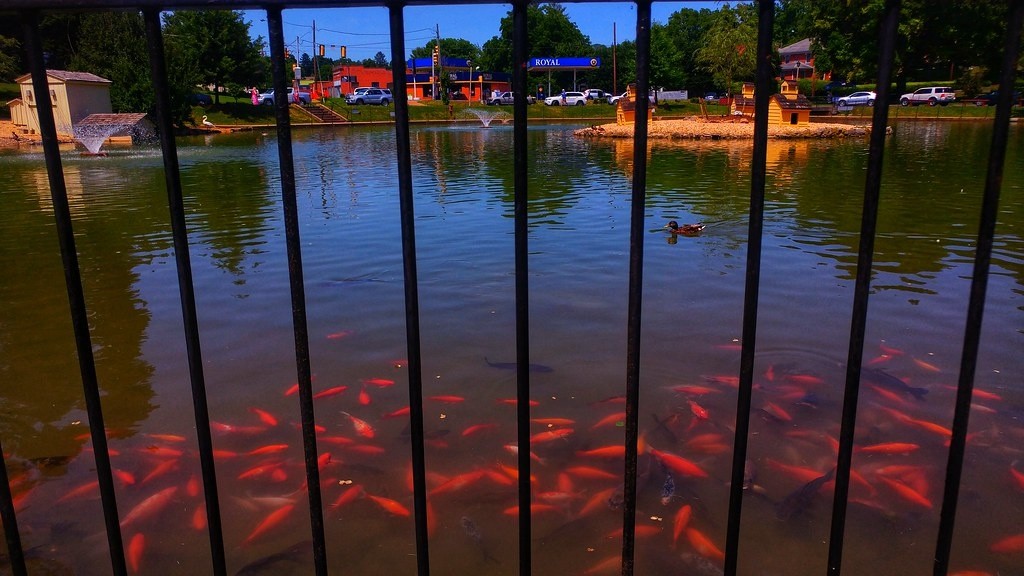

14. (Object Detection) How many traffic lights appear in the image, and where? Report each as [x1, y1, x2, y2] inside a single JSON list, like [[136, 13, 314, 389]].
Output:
[[433, 53, 437, 62], [293, 64, 296, 71], [320, 45, 325, 56], [286, 48, 290, 58], [435, 46, 438, 52], [341, 46, 346, 58]]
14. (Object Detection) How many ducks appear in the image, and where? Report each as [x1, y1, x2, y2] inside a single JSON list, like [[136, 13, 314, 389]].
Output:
[[663, 221, 707, 237]]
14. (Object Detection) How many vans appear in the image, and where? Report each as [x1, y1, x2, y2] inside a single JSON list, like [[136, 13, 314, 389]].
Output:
[[354, 87, 374, 95]]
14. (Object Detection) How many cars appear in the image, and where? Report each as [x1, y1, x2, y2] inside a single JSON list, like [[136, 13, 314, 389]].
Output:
[[719, 93, 728, 99], [609, 92, 654, 105], [544, 92, 587, 107], [584, 89, 612, 101], [832, 92, 876, 107], [490, 92, 536, 106], [973, 89, 1020, 107], [705, 92, 718, 100]]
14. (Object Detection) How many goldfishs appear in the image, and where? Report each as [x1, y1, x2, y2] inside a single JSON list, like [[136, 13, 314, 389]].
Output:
[[1, 329, 1023, 575]]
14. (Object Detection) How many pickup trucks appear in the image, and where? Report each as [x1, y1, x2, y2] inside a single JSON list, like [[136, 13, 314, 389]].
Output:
[[258, 87, 311, 106]]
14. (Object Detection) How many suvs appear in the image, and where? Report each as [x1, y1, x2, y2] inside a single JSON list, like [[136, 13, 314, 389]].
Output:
[[346, 88, 393, 106], [900, 87, 957, 106]]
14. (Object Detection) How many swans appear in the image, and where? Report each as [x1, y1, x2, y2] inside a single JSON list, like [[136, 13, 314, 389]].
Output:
[[202, 115, 216, 131]]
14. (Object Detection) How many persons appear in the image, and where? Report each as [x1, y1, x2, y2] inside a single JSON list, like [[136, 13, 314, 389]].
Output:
[[561, 89, 568, 106], [295, 90, 299, 103], [251, 87, 258, 105]]
